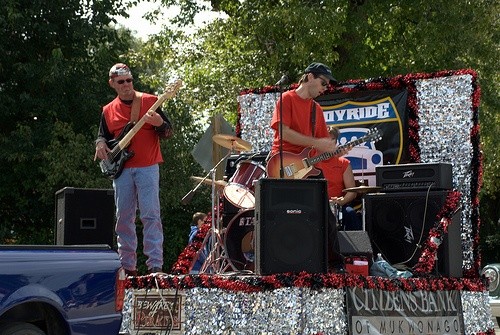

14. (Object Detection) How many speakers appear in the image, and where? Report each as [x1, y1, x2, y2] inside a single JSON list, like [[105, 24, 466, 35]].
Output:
[[362, 190, 462, 279], [253, 178, 329, 272]]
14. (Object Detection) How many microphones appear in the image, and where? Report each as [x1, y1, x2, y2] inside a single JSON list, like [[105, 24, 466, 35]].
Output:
[[276, 74, 289, 86]]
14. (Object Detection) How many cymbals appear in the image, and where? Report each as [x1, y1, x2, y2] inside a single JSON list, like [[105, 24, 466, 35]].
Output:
[[341, 185, 384, 193], [209, 133, 252, 151], [190, 174, 228, 188]]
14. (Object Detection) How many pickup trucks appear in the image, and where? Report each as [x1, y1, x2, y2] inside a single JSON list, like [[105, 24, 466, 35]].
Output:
[[0, 240, 127, 335]]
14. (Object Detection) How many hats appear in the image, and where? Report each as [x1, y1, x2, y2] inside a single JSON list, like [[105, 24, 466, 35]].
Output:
[[305, 62, 338, 86], [109, 63, 131, 78]]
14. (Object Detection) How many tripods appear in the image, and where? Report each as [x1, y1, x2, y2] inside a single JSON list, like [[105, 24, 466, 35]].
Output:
[[193, 144, 232, 275]]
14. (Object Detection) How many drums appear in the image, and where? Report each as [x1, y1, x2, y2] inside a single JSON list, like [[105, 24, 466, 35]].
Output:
[[223, 158, 269, 210], [223, 210, 255, 276]]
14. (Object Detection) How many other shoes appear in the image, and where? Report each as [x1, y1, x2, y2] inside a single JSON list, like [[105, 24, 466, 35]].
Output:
[[126, 268, 137, 276], [148, 267, 163, 273]]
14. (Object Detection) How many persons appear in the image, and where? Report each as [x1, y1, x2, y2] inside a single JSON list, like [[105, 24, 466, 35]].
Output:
[[310, 126, 360, 231], [94, 63, 173, 276], [270, 62, 349, 179], [188, 213, 208, 274]]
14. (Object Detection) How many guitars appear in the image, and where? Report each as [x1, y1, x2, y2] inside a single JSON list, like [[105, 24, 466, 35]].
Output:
[[265, 125, 385, 180], [96, 76, 185, 182]]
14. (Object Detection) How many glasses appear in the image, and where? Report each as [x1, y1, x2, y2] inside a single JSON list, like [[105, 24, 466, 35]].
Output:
[[312, 73, 329, 89], [111, 79, 133, 84]]
